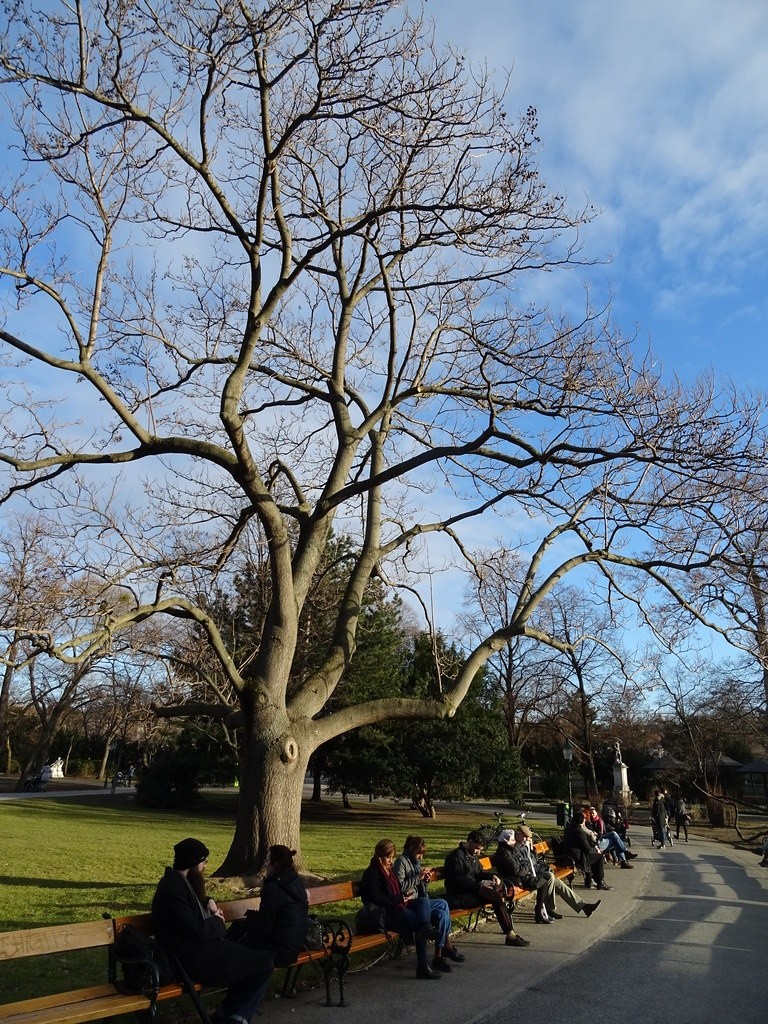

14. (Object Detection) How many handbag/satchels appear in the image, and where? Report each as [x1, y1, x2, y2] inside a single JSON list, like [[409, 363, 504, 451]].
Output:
[[354, 902, 386, 936], [682, 814, 691, 820], [303, 924, 323, 951]]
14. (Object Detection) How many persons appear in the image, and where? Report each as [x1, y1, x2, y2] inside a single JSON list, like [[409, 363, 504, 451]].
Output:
[[359, 839, 441, 979], [40, 762, 52, 793], [151, 837, 274, 1024], [50, 756, 64, 772], [649, 786, 689, 849], [394, 835, 465, 971], [751, 841, 768, 867], [444, 830, 530, 947], [495, 824, 601, 924], [565, 804, 638, 890], [227, 845, 309, 966], [128, 765, 136, 787], [614, 737, 623, 763]]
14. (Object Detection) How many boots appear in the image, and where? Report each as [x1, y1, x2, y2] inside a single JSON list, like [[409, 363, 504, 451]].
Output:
[[416, 961, 442, 980], [422, 922, 441, 941]]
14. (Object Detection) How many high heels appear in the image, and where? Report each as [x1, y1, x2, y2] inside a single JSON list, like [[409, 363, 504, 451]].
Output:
[[535, 914, 555, 924]]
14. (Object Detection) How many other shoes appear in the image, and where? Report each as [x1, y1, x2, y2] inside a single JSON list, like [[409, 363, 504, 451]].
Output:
[[441, 943, 465, 962], [547, 910, 564, 919], [657, 844, 665, 849], [624, 851, 638, 860], [674, 836, 679, 840], [621, 860, 634, 869], [431, 956, 451, 972], [505, 934, 530, 947], [686, 839, 689, 842], [596, 880, 613, 890], [582, 900, 602, 917], [758, 856, 768, 867], [750, 847, 763, 856]]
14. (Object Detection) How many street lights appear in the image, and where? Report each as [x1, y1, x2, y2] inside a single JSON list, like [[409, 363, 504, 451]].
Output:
[[563, 742, 575, 820]]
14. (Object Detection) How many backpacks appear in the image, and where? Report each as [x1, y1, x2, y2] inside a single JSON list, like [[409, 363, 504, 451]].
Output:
[[107, 922, 178, 996]]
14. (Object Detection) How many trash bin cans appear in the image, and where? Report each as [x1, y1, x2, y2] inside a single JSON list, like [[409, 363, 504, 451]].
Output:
[[556, 803, 570, 826]]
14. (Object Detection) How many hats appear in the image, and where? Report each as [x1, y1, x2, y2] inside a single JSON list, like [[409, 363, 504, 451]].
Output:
[[172, 837, 210, 870], [497, 828, 516, 845], [517, 825, 533, 838]]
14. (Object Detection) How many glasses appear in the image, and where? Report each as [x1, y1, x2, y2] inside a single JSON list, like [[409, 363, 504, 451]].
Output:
[[203, 860, 209, 865]]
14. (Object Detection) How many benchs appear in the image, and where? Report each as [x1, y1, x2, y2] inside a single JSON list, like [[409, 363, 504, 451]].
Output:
[[0, 841, 581, 1024]]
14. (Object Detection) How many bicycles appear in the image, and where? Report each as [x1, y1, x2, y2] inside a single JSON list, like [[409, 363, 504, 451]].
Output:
[[477, 806, 546, 860]]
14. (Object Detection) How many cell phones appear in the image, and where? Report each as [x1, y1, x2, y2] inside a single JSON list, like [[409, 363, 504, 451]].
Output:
[[425, 868, 436, 876]]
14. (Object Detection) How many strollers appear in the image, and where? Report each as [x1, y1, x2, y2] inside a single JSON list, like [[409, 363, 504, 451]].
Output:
[[649, 819, 674, 846], [610, 810, 631, 848]]
